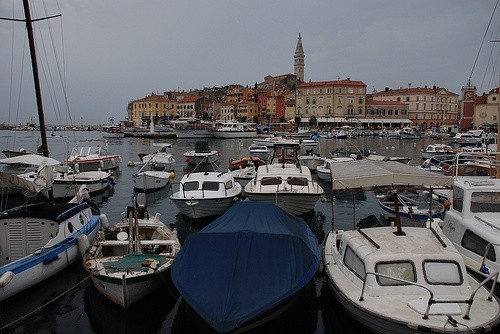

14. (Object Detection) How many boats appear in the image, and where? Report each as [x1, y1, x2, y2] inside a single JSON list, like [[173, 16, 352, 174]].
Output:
[[322, 185, 500, 334], [442, 176, 500, 282], [170, 200, 321, 334], [84, 192, 181, 309], [3, 120, 500, 220], [376, 186, 450, 228]]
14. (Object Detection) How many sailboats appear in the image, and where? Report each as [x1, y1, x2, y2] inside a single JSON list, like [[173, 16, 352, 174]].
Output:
[[0, 0, 100, 299]]
